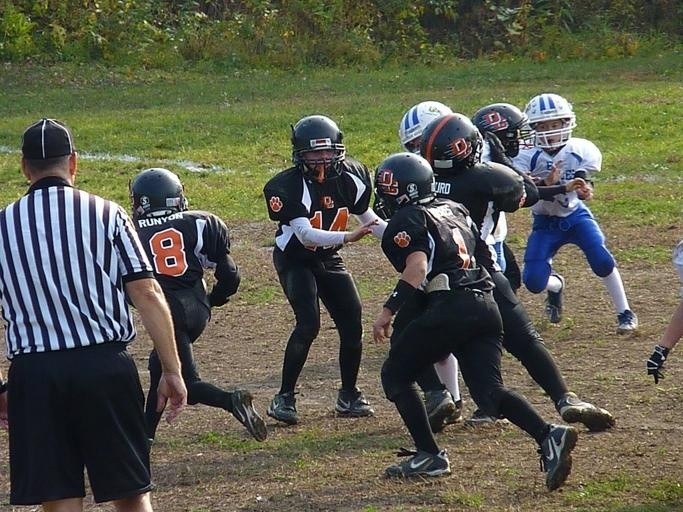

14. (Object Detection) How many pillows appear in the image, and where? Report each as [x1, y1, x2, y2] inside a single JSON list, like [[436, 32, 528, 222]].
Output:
[[372, 152, 436, 221], [419, 112, 483, 175], [290, 115, 345, 185], [471, 103, 535, 158], [523, 93, 577, 148], [129, 168, 188, 220], [398, 100, 452, 156]]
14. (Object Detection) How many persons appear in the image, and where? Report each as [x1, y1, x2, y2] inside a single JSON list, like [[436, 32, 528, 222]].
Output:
[[1, 118, 188, 511], [373, 153, 579, 489], [381, 102, 615, 432], [513, 95, 636, 334], [648, 239, 683, 384], [120, 168, 267, 442], [265, 115, 389, 424]]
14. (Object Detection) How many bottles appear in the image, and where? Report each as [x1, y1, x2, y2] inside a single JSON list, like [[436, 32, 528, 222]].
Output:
[[21, 117, 75, 159]]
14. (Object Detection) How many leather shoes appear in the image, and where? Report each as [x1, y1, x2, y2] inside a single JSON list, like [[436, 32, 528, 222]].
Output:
[[645, 344, 669, 384]]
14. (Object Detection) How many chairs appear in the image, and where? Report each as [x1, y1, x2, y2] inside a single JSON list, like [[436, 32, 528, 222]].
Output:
[[266, 391, 299, 425], [542, 273, 565, 323], [554, 391, 614, 431], [384, 447, 451, 479], [230, 390, 266, 443], [536, 424, 578, 491], [615, 309, 637, 335], [334, 387, 374, 417], [422, 388, 509, 434]]
